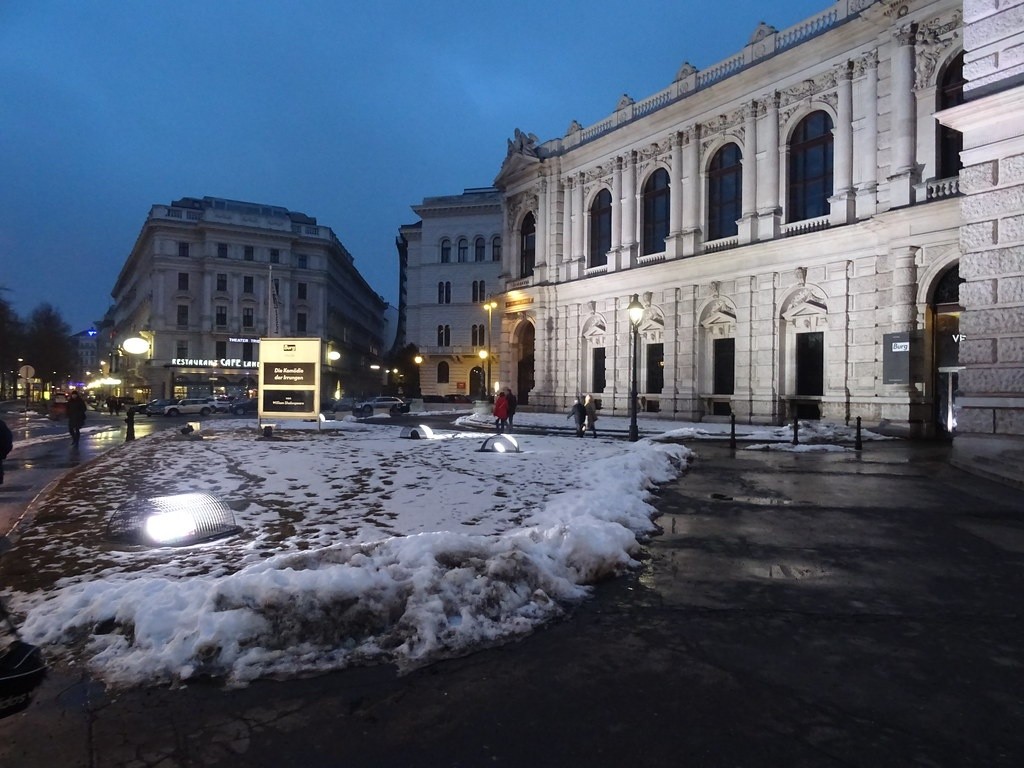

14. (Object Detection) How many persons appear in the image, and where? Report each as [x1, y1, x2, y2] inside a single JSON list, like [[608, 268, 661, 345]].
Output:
[[107, 395, 124, 416], [66, 389, 88, 448], [584, 394, 598, 438], [0, 420, 13, 485], [505, 388, 517, 429], [567, 396, 586, 438], [494, 392, 509, 433]]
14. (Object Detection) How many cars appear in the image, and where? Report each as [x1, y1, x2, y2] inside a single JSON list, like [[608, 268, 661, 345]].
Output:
[[145, 399, 170, 417], [445, 394, 472, 403], [229, 396, 259, 416], [322, 398, 337, 410], [333, 396, 356, 411], [354, 396, 404, 413], [131, 404, 146, 415], [419, 394, 446, 403], [204, 397, 232, 413]]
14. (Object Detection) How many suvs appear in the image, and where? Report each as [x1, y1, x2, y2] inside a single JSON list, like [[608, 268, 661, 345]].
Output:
[[163, 399, 215, 416]]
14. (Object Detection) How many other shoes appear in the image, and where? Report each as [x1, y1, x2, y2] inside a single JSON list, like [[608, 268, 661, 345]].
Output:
[[71, 438, 75, 444], [75, 441, 78, 445]]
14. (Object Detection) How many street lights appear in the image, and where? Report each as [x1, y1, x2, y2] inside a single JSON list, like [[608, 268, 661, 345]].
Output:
[[485, 290, 497, 403], [627, 293, 644, 443], [479, 345, 486, 403]]
